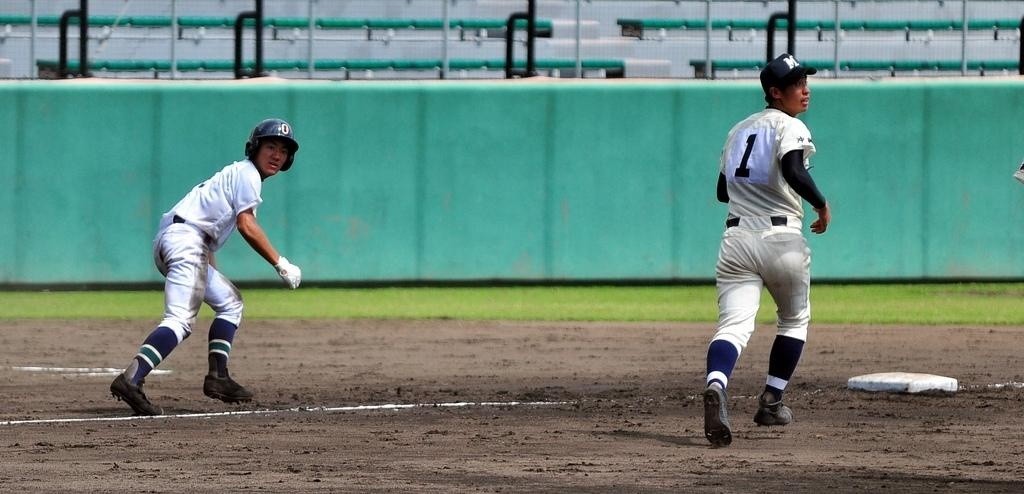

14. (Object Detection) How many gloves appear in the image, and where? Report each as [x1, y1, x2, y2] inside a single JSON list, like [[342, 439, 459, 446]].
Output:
[[275, 257, 301, 290]]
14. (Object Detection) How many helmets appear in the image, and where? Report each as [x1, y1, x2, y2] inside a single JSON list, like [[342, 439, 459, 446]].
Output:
[[246, 119, 299, 171]]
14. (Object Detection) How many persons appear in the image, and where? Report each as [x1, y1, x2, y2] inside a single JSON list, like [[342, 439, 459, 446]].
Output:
[[700, 48, 834, 447], [108, 118, 305, 415]]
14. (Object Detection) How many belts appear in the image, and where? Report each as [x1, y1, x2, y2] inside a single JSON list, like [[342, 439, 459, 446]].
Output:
[[726, 217, 787, 228], [172, 215, 213, 245]]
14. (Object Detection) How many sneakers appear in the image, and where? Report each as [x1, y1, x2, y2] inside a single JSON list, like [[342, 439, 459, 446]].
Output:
[[203, 375, 253, 402], [753, 394, 793, 425], [703, 385, 732, 446], [110, 374, 163, 416]]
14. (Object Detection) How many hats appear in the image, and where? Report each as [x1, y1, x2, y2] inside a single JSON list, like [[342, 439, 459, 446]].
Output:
[[760, 54, 817, 80]]
